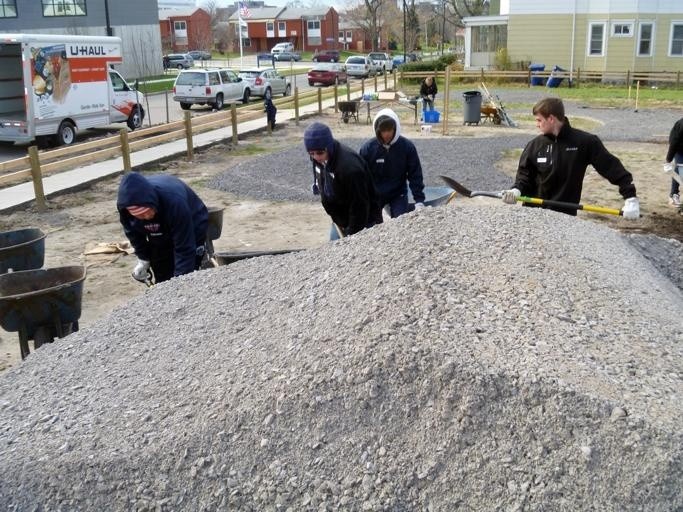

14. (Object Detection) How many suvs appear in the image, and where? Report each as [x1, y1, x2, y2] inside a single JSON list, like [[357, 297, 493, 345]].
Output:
[[367, 52, 392, 75], [171, 68, 249, 110]]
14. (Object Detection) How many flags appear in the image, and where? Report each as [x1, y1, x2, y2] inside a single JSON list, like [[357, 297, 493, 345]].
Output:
[[239, 2, 252, 19]]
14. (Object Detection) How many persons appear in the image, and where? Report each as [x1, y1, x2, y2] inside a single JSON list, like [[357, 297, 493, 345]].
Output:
[[117, 173, 210, 283], [304, 120, 379, 238], [357, 109, 426, 221], [501, 97, 640, 222], [420, 77, 437, 111], [663, 117, 683, 208]]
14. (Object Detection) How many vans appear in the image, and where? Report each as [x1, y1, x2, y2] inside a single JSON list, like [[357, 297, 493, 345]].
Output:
[[0, 34, 147, 144]]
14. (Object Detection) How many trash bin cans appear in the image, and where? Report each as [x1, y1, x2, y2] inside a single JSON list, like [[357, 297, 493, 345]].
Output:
[[462, 90, 482, 126], [528, 64, 545, 86], [546, 65, 566, 88]]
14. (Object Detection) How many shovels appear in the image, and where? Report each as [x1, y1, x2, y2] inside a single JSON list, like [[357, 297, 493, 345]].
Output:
[[438, 174, 643, 218]]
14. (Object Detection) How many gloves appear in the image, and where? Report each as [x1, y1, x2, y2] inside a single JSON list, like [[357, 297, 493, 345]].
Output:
[[132, 255, 150, 280], [623, 197, 640, 221], [663, 162, 672, 174], [501, 187, 521, 204], [414, 202, 424, 209]]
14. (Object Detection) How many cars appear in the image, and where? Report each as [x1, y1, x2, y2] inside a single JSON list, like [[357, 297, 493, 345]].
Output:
[[163, 53, 194, 69], [307, 63, 347, 85], [311, 50, 339, 62], [236, 69, 291, 100], [390, 56, 409, 68], [272, 52, 300, 62], [187, 51, 211, 60], [270, 42, 294, 55], [343, 56, 375, 77]]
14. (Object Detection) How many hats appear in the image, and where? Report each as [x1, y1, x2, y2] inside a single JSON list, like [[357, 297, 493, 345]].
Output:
[[126, 204, 152, 217], [304, 122, 334, 161]]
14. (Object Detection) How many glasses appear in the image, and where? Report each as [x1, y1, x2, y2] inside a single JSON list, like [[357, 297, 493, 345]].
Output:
[[308, 151, 325, 155]]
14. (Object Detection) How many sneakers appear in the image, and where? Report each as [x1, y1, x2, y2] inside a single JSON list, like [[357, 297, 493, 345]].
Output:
[[668, 193, 683, 208]]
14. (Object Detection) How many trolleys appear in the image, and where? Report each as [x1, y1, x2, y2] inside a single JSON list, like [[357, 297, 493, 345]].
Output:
[[335, 99, 360, 122]]
